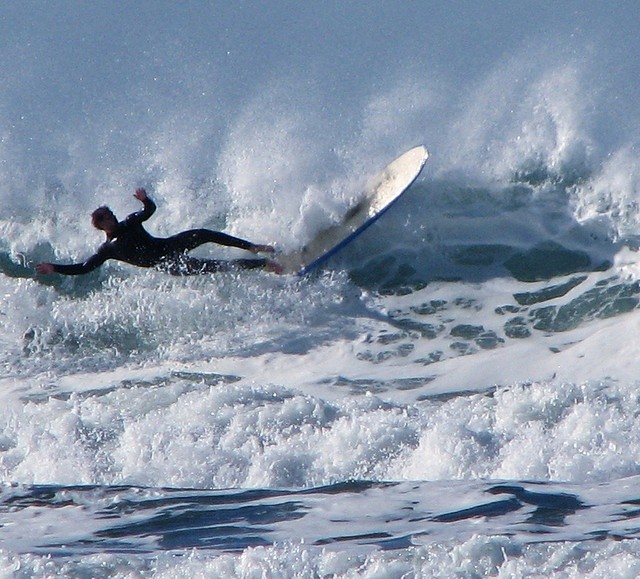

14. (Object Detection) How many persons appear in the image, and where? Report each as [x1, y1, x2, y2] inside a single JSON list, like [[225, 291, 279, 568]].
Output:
[[35, 186, 285, 277]]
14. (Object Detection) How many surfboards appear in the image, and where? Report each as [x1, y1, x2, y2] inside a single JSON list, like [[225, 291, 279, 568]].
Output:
[[257, 144, 430, 276]]
